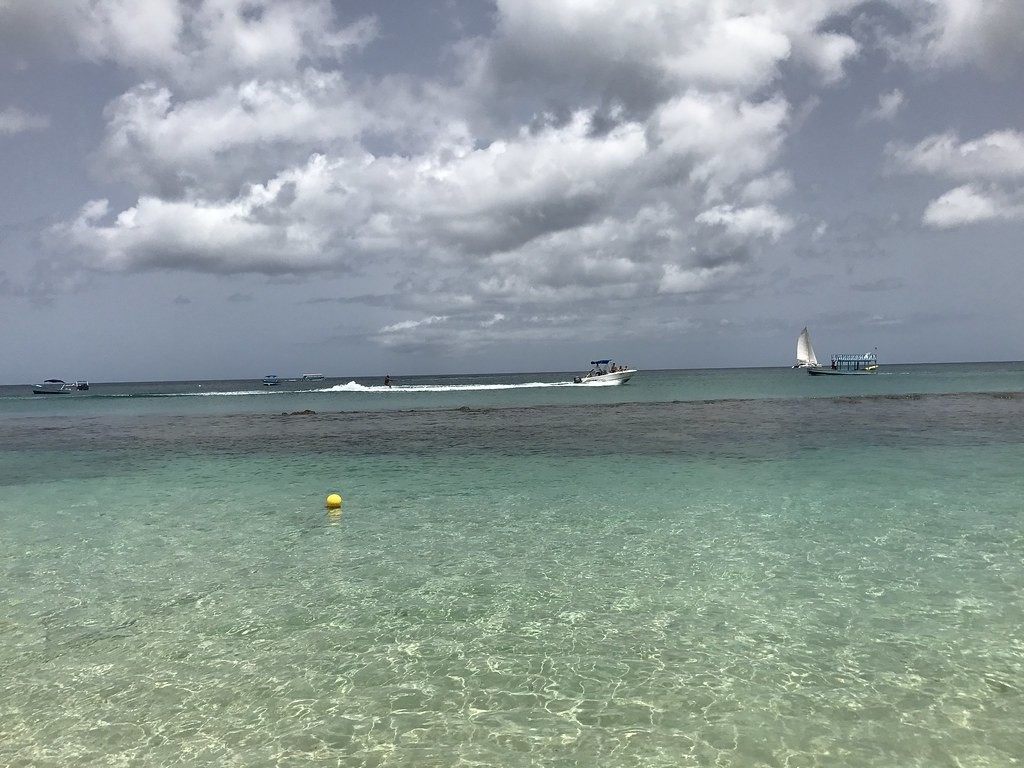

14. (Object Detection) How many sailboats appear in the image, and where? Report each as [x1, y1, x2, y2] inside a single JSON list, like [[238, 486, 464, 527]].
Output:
[[791, 325, 823, 369]]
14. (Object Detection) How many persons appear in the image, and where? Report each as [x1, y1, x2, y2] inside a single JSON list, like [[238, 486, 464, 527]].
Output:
[[595, 363, 630, 375], [384, 374, 396, 389]]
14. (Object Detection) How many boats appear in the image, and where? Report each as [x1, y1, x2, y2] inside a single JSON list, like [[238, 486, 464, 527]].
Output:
[[807, 346, 880, 376], [300, 373, 325, 383], [263, 374, 281, 386], [573, 359, 637, 386], [30, 378, 88, 395]]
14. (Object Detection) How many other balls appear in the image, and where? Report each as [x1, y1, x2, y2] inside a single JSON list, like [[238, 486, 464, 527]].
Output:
[[325, 493, 343, 508]]
